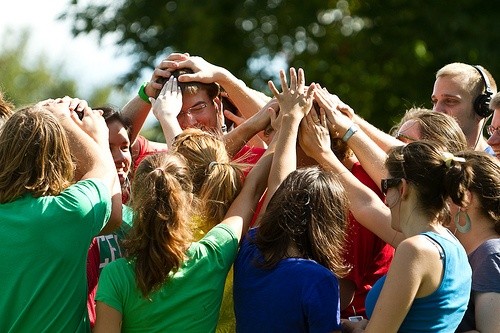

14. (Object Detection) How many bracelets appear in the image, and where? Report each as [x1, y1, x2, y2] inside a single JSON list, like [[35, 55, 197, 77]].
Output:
[[138, 82, 159, 104], [342, 124, 360, 143]]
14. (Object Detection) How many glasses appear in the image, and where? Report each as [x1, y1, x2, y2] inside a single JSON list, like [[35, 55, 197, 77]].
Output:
[[396, 133, 416, 143], [381, 178, 408, 194], [177, 95, 216, 121], [487, 125, 500, 135]]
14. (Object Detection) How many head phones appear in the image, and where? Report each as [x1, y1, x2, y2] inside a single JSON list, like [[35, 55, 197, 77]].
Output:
[[474, 67, 494, 118]]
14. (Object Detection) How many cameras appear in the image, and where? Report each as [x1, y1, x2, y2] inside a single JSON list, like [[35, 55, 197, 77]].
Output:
[[348, 316, 363, 322]]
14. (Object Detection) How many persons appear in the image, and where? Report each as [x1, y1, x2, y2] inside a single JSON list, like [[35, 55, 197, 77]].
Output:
[[92, 107, 282, 333], [298, 105, 473, 333], [0, 52, 500, 328], [0, 102, 116, 333], [314, 91, 500, 333], [233, 67, 354, 333]]
